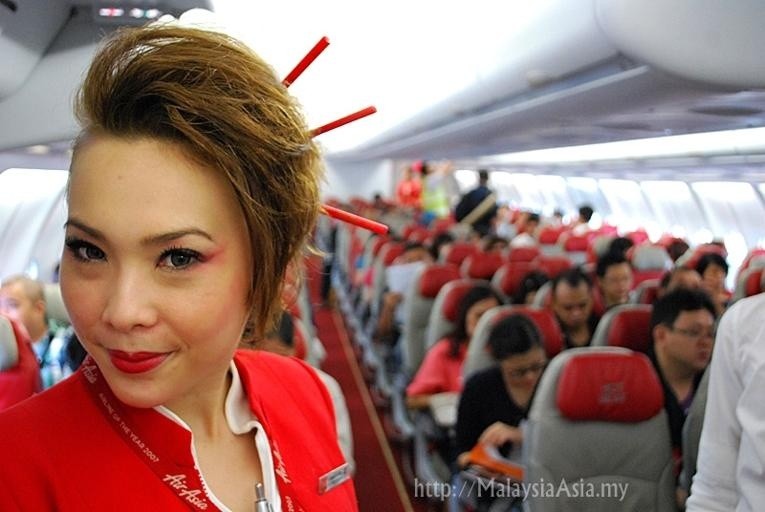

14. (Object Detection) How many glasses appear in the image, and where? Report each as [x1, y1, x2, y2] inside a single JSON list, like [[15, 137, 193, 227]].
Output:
[[510, 362, 542, 376], [670, 323, 714, 339]]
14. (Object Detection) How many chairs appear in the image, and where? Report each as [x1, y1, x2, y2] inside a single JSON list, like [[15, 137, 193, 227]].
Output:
[[340, 200, 765, 512]]
